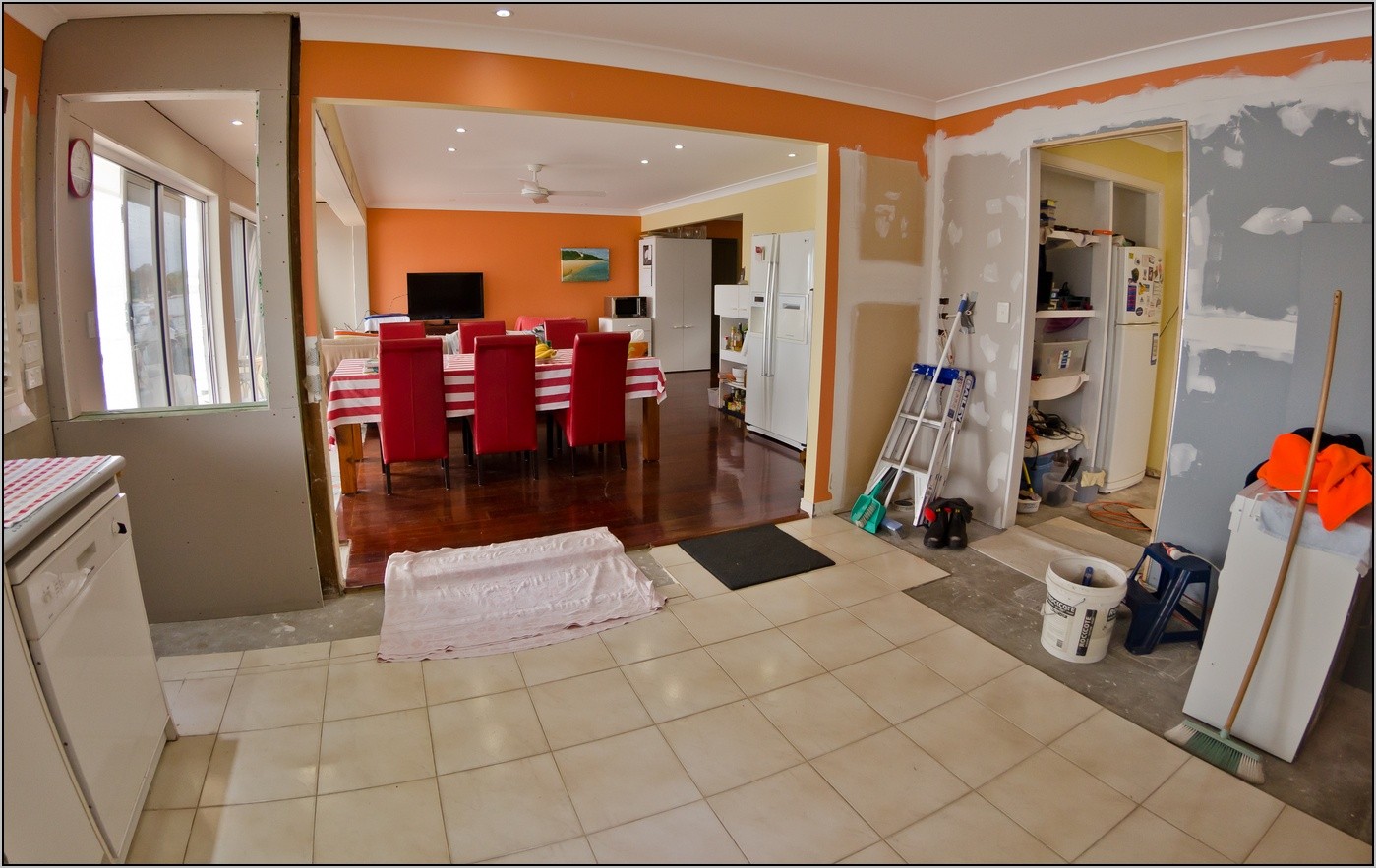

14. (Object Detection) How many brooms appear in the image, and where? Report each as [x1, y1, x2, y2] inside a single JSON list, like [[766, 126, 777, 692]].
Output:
[[1160, 289, 1342, 786], [878, 293, 968, 540]]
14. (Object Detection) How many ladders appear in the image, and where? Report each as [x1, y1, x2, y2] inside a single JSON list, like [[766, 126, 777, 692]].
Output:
[[864, 363, 975, 527]]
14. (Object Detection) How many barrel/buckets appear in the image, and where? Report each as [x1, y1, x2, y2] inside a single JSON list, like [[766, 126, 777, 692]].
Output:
[[1041, 473, 1079, 508], [1073, 467, 1103, 503], [1040, 555, 1128, 665], [1017, 497, 1041, 513], [1031, 454, 1068, 496]]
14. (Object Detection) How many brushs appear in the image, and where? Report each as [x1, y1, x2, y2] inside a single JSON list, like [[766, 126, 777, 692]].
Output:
[[855, 480, 884, 528]]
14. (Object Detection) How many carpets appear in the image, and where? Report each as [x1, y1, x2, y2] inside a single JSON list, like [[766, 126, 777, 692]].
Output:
[[967, 516, 1147, 584], [678, 522, 836, 590]]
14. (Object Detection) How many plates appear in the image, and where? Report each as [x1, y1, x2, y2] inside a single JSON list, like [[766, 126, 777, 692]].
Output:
[[535, 357, 556, 364]]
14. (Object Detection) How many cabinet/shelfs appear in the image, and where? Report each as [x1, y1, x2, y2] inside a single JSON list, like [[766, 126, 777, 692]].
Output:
[[639, 238, 712, 372], [1023, 231, 1100, 457], [714, 285, 751, 408], [599, 317, 652, 356]]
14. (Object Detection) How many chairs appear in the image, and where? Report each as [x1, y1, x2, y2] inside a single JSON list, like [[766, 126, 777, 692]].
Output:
[[458, 321, 540, 487], [543, 319, 627, 477], [378, 322, 451, 494]]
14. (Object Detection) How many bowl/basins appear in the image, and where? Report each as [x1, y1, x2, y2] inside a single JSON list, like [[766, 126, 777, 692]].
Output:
[[731, 367, 745, 383]]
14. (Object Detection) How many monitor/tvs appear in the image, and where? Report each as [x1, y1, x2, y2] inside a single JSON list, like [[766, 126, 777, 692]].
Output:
[[407, 273, 484, 327]]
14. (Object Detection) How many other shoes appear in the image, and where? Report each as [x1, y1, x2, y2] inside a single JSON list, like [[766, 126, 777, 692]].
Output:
[[948, 509, 968, 550], [923, 506, 947, 549]]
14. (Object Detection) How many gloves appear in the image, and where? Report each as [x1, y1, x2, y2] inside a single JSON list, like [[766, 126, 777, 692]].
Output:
[[922, 497, 975, 523]]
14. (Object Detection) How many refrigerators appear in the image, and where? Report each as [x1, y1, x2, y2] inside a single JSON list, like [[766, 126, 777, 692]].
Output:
[[744, 230, 816, 451], [1092, 244, 1165, 493]]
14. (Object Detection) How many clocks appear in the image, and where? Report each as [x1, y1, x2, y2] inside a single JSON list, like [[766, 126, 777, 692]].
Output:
[[68, 138, 94, 198]]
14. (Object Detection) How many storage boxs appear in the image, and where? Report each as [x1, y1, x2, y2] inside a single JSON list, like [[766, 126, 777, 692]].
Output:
[[708, 387, 719, 407], [1034, 337, 1089, 378]]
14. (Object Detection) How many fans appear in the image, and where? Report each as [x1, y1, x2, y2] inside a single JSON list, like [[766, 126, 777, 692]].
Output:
[[464, 164, 605, 205]]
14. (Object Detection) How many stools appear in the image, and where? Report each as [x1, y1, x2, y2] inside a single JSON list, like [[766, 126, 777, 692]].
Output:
[[1121, 541, 1212, 656]]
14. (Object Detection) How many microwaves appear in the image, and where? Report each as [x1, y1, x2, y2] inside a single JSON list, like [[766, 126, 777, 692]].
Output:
[[604, 296, 648, 318]]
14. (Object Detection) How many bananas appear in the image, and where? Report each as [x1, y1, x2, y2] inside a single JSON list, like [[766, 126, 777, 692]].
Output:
[[535, 344, 557, 359]]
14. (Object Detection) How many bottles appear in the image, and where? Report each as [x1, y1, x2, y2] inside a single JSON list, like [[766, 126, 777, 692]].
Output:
[[724, 387, 745, 411], [1059, 449, 1071, 465], [724, 322, 749, 353]]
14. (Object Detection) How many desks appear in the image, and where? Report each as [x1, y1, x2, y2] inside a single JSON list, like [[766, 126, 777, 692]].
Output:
[[326, 348, 667, 495], [514, 315, 576, 331]]
